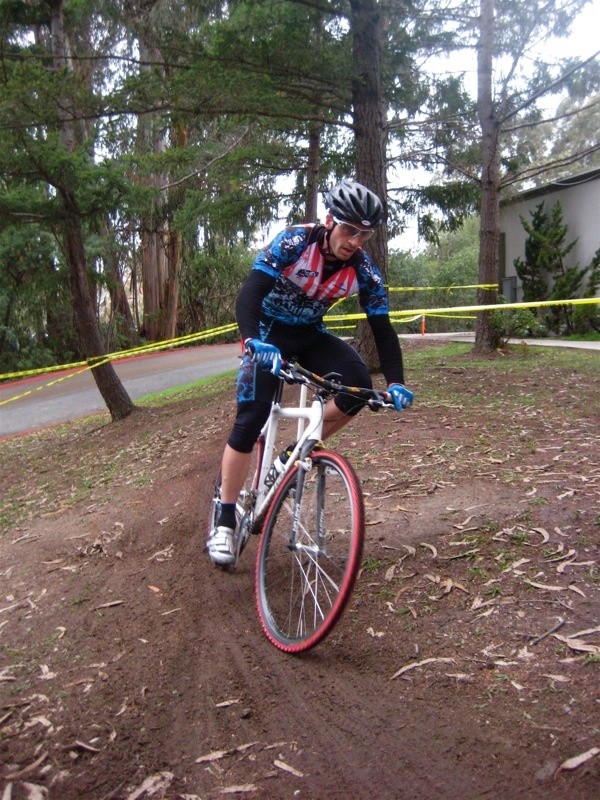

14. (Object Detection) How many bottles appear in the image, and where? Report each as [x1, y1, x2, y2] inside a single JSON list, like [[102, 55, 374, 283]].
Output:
[[260, 443, 297, 497]]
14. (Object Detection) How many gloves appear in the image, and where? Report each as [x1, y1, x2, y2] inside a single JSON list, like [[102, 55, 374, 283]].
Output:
[[384, 383, 414, 411], [244, 337, 281, 376]]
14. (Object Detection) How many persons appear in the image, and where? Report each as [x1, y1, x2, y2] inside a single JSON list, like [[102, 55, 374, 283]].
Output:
[[208, 180, 415, 565]]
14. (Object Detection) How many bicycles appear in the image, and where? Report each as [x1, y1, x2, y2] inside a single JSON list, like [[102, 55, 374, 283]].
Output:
[[207, 346, 404, 654]]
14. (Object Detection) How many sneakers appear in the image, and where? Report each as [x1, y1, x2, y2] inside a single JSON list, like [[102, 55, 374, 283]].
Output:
[[207, 525, 235, 564], [273, 451, 290, 472]]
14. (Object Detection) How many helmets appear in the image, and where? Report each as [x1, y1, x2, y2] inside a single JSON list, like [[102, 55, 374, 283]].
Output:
[[327, 181, 383, 231]]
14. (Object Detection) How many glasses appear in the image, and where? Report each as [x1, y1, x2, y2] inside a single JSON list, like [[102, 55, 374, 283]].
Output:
[[329, 213, 375, 240]]
